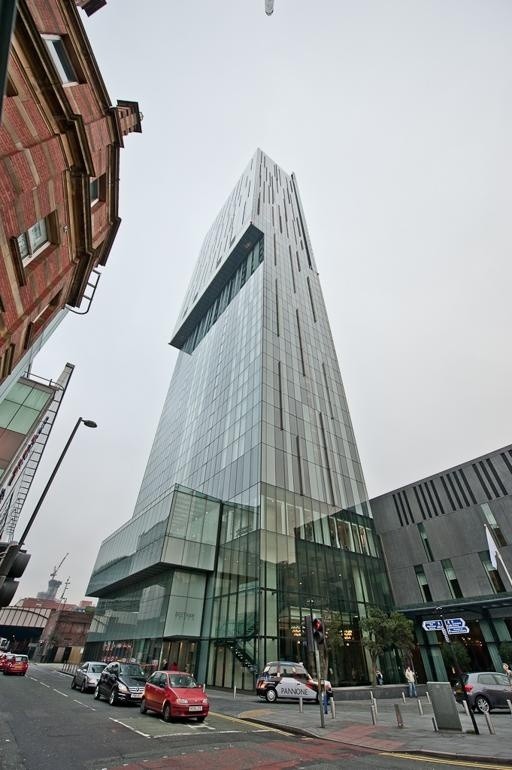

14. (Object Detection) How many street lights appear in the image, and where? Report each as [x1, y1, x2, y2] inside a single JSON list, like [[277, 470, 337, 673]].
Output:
[[0, 417, 97, 589]]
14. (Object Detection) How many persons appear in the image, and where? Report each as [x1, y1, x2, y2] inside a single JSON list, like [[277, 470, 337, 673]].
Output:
[[309, 672, 331, 714], [405, 666, 418, 698], [501, 662, 511, 691]]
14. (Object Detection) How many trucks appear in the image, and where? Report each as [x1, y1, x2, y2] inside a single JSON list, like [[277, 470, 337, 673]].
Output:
[[0, 652, 28, 676]]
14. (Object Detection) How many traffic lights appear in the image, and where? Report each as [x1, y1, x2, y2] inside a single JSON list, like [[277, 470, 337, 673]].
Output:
[[314, 618, 324, 644], [0, 542, 31, 610]]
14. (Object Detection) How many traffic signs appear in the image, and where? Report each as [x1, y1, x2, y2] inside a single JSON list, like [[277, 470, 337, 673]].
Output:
[[422, 618, 469, 635]]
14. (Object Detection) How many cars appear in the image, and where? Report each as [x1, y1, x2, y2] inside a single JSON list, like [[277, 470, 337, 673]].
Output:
[[454, 672, 512, 714], [71, 661, 209, 722], [256, 661, 333, 703]]
